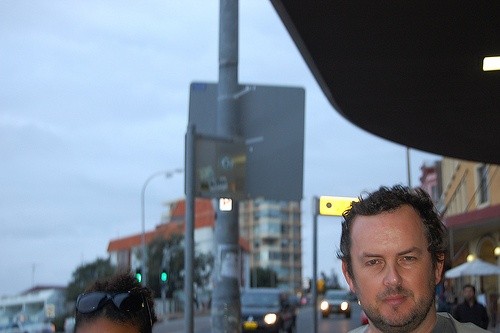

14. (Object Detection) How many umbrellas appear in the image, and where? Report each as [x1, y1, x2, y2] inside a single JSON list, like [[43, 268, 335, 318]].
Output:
[[444, 257, 500, 291]]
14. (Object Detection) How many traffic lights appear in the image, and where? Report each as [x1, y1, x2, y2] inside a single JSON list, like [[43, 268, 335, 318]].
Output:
[[136, 272, 142, 283], [159, 269, 168, 284]]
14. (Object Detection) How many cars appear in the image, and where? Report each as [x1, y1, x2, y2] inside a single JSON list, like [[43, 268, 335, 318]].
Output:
[[321, 288, 353, 319], [240, 288, 298, 333]]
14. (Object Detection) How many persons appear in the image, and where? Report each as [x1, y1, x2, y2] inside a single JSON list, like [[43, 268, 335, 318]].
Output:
[[451, 285, 489, 328], [335, 185, 490, 333], [72, 271, 157, 332]]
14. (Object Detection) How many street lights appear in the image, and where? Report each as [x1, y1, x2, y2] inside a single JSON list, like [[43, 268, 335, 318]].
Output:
[[140, 168, 184, 285]]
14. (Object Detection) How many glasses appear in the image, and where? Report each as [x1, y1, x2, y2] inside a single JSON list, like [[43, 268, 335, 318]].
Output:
[[76, 291, 152, 327]]
